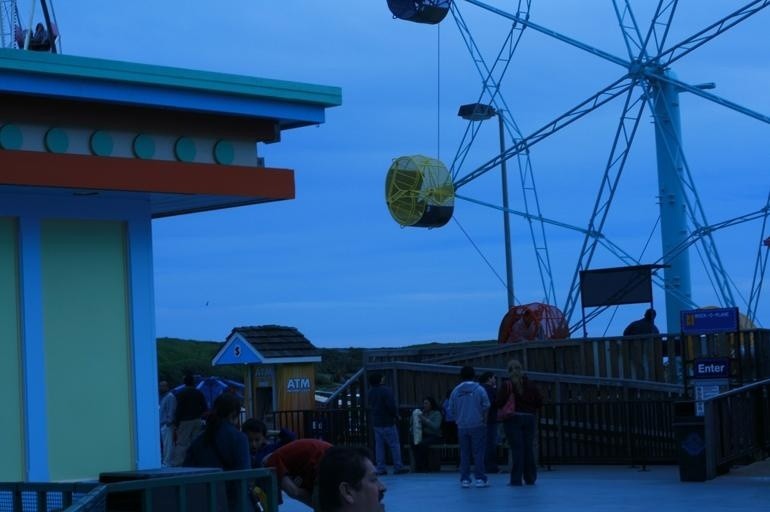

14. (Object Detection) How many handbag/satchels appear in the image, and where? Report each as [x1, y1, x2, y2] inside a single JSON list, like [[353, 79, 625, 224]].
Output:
[[496, 393, 516, 420]]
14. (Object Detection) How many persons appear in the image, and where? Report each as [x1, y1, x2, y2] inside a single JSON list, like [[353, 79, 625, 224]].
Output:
[[508, 310, 534, 342], [492, 359, 543, 487], [478, 370, 501, 474], [448, 365, 491, 488], [33, 23, 48, 45], [623, 308, 660, 336]]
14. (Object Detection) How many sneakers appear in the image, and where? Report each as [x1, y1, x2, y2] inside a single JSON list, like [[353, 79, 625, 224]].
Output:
[[475, 479, 489, 487], [462, 479, 471, 488]]
